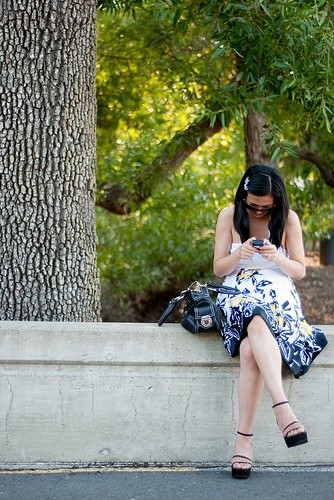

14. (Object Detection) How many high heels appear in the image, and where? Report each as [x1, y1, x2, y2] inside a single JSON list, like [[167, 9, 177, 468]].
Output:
[[272, 401, 308, 448], [231, 431, 253, 479]]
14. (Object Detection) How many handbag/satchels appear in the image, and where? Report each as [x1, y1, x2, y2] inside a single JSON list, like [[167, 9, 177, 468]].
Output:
[[157, 281, 241, 333]]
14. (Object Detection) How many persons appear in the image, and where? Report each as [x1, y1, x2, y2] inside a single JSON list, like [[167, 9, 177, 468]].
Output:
[[213, 165, 327, 480]]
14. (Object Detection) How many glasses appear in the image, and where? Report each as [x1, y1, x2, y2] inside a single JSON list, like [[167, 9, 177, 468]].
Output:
[[243, 199, 276, 212]]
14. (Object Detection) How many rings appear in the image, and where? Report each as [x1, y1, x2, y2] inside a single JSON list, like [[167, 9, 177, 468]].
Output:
[[267, 254, 271, 259]]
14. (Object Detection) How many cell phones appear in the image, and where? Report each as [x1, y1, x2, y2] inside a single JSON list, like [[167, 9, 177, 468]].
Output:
[[252, 239, 265, 247]]
